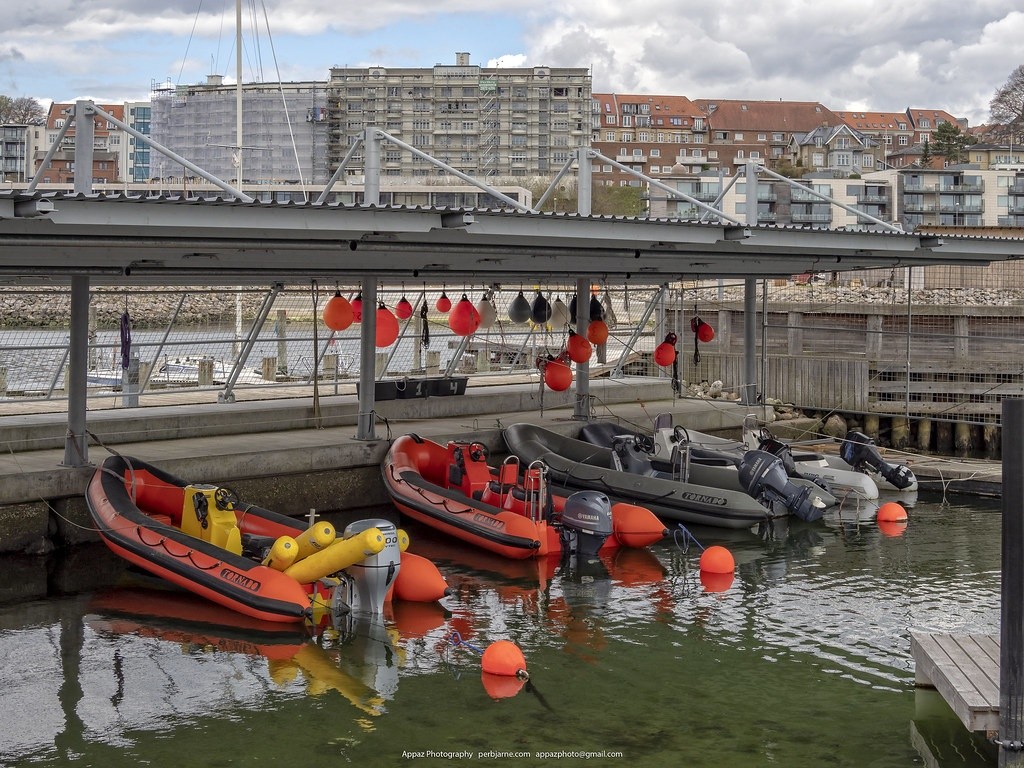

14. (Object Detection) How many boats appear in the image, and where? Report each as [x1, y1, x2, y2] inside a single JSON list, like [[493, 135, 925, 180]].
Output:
[[651, 413, 917, 496], [506, 422, 841, 528], [383, 433, 671, 555], [87, 453, 451, 622], [582, 422, 843, 517], [76, 355, 358, 387]]
